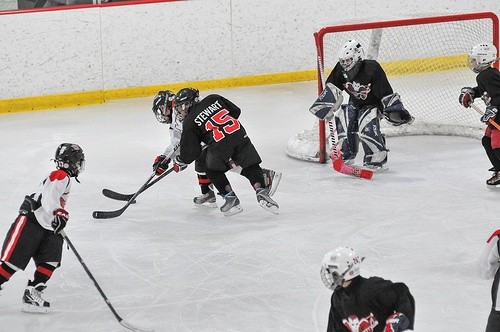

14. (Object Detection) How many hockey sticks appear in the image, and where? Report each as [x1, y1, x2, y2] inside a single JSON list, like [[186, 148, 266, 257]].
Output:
[[102, 165, 178, 201], [60, 227, 151, 332], [467, 99, 500, 131], [91, 139, 181, 220], [317, 53, 373, 180]]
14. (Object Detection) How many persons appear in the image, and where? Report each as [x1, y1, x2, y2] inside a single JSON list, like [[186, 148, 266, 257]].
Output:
[[308, 39, 411, 169], [485, 239, 500, 332], [320, 245, 415, 332], [459, 43, 500, 185], [173, 87, 280, 212], [0, 143, 86, 307], [152, 89, 276, 205]]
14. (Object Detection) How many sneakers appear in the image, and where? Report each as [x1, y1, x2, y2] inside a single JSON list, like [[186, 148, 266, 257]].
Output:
[[329, 149, 356, 166], [193, 184, 218, 209], [21, 279, 52, 314], [361, 162, 390, 173], [262, 168, 283, 197], [255, 188, 280, 215], [216, 190, 244, 217], [486, 170, 500, 191]]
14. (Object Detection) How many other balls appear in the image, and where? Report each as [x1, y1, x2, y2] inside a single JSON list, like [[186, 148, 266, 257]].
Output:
[[128, 200, 136, 204]]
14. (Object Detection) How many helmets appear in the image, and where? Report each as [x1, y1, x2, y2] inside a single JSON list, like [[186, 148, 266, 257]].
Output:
[[466, 42, 497, 68], [173, 87, 200, 123], [151, 90, 176, 125], [338, 39, 364, 79], [322, 246, 361, 282], [54, 143, 86, 177]]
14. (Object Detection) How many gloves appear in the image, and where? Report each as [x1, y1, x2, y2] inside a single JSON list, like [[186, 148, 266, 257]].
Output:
[[173, 155, 188, 173], [383, 312, 410, 332], [458, 86, 475, 108], [51, 209, 69, 234], [480, 107, 499, 126], [152, 155, 171, 176]]
